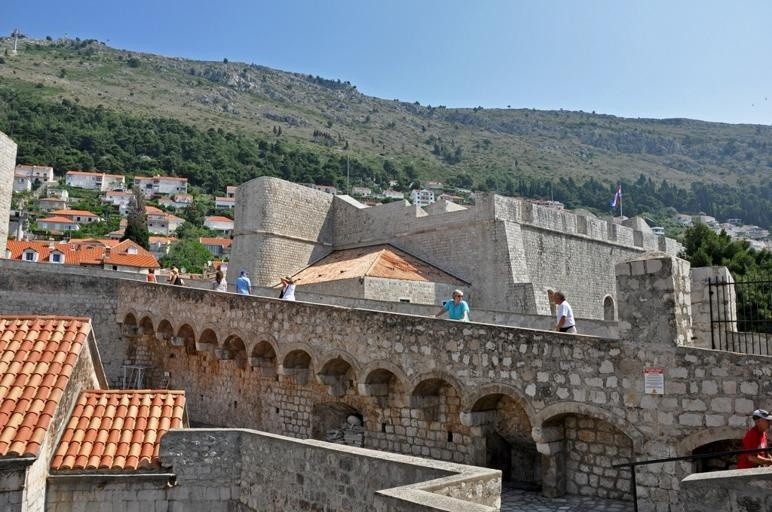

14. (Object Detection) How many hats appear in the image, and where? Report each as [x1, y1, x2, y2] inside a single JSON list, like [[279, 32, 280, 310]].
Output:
[[752, 410, 772, 420], [173, 268, 178, 274]]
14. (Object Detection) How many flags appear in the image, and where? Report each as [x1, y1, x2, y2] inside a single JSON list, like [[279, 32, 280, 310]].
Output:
[[609, 183, 621, 209]]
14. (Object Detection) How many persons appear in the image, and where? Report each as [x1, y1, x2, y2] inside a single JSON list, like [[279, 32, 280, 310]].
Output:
[[145, 268, 157, 283], [553, 292, 576, 333], [281, 275, 296, 300], [167, 263, 185, 286], [235, 269, 252, 294], [212, 271, 227, 292], [434, 289, 471, 321], [735, 409, 772, 469]]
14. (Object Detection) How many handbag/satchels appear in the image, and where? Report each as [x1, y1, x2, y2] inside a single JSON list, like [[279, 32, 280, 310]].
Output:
[[180, 279, 185, 285], [279, 284, 290, 298]]
